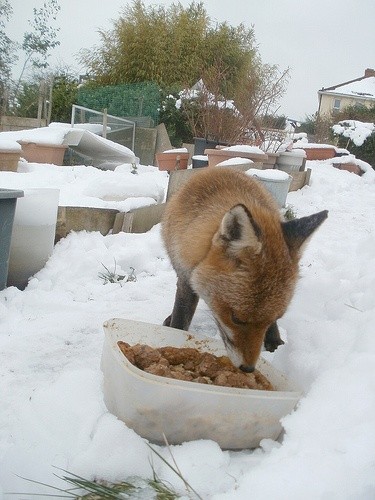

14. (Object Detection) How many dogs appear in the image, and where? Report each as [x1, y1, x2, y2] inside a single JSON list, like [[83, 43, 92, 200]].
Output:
[[161, 168, 329, 373]]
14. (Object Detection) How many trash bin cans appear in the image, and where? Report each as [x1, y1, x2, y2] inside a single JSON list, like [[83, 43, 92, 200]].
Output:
[[0, 187, 26, 291]]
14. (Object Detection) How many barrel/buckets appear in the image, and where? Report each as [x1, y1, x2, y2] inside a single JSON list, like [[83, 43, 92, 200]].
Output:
[[253, 172, 293, 210], [7, 186, 60, 291]]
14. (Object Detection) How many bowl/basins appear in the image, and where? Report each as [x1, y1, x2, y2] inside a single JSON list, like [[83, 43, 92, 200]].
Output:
[[101, 318, 297, 450]]
